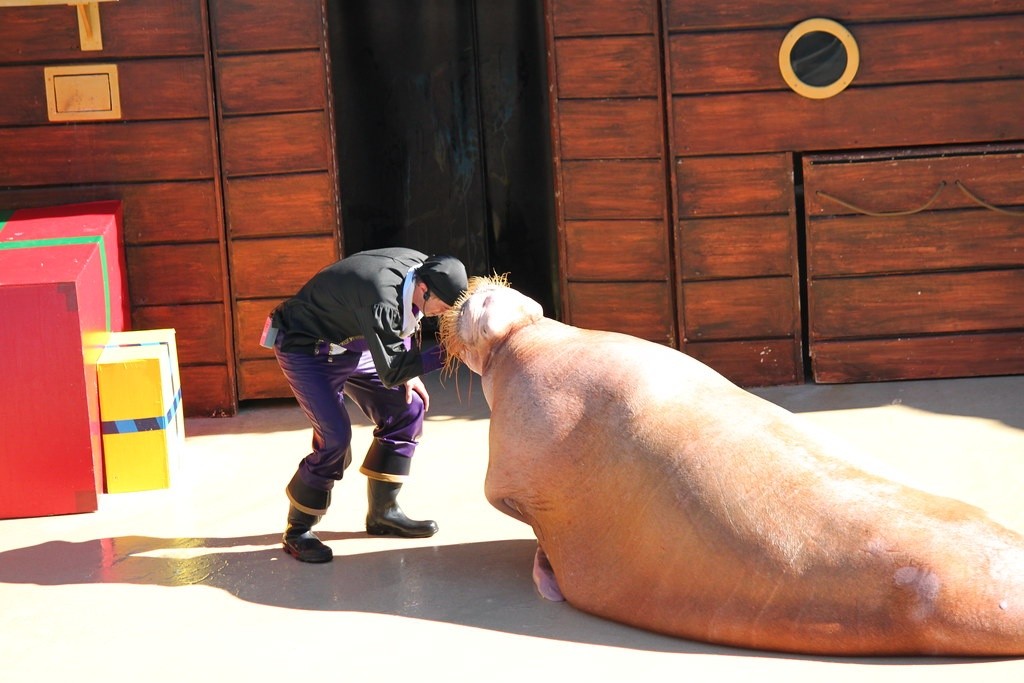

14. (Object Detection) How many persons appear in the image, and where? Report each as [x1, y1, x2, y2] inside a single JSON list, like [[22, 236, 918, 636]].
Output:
[[268, 247, 470, 563]]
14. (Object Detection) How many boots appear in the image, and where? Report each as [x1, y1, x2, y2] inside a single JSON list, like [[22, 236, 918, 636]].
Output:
[[360, 440, 439, 538], [282, 471, 333, 563]]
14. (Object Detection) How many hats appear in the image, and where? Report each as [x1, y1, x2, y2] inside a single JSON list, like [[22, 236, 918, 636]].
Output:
[[416, 255, 468, 307]]
